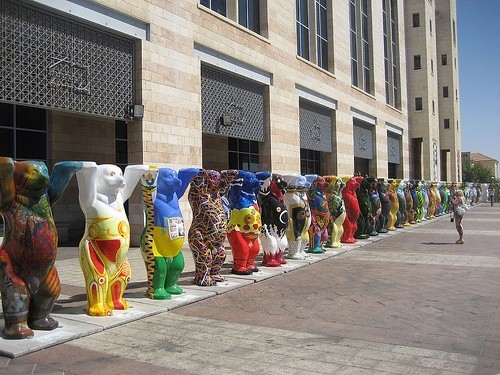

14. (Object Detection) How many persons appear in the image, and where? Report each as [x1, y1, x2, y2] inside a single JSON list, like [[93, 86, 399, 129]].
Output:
[[490, 187, 495, 207], [451, 190, 465, 244]]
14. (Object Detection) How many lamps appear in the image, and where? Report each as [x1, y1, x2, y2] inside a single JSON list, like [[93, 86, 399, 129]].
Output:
[[123, 104, 144, 120], [215, 115, 231, 133]]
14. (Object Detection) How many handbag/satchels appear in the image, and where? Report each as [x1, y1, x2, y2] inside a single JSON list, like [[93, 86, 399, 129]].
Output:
[[455, 198, 470, 215]]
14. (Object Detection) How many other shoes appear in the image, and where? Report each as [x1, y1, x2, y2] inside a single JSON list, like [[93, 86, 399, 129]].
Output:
[[456, 239, 464, 244]]
